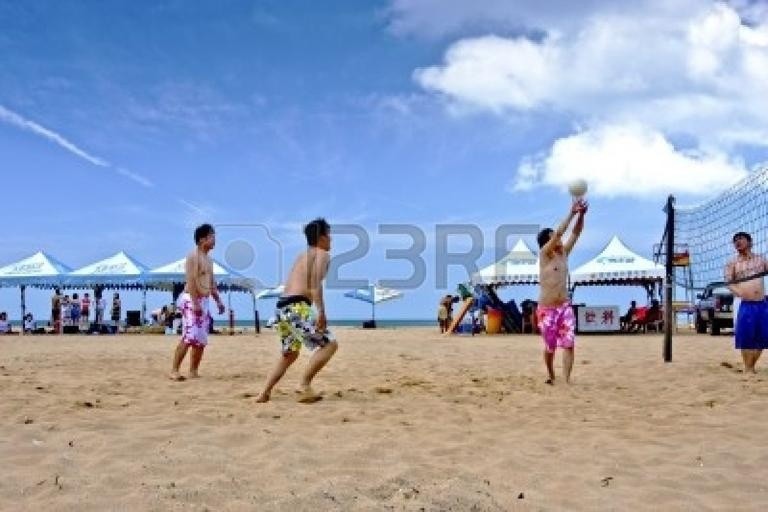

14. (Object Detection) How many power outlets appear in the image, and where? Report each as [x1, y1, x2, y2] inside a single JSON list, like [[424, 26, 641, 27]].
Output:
[[695, 281, 768, 334]]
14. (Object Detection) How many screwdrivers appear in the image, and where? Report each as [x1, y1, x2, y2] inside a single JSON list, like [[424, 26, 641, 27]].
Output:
[[569, 177, 587, 197]]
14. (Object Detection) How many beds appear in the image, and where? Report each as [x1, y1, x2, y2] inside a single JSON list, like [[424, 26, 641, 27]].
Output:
[[343, 282, 404, 321]]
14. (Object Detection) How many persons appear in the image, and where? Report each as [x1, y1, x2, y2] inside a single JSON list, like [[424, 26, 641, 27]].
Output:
[[109, 292, 121, 333], [167, 303, 177, 328], [451, 295, 460, 311], [61, 295, 72, 326], [81, 292, 91, 322], [71, 292, 81, 326], [24, 313, 36, 331], [437, 294, 452, 334], [150, 304, 168, 323], [0, 312, 9, 332], [254, 217, 338, 404], [537, 196, 591, 384], [95, 294, 106, 324], [628, 300, 662, 333], [620, 300, 639, 331], [168, 223, 227, 382], [52, 289, 62, 333], [723, 232, 768, 374]]
